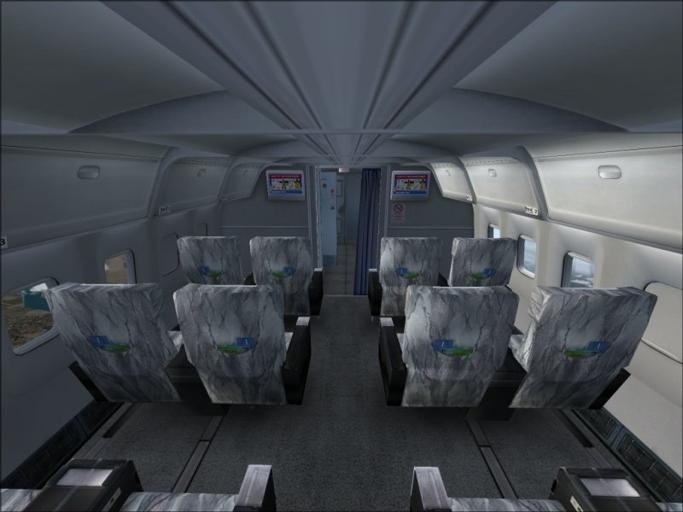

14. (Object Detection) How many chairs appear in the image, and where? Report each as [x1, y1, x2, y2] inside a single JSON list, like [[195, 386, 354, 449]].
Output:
[[173, 236, 252, 294], [43, 280, 185, 438], [372, 280, 522, 414], [59, 455, 281, 512], [1, 452, 102, 510], [368, 235, 454, 326], [508, 282, 660, 413], [172, 278, 317, 404], [406, 460, 681, 512], [449, 236, 523, 329], [244, 237, 323, 320]]
